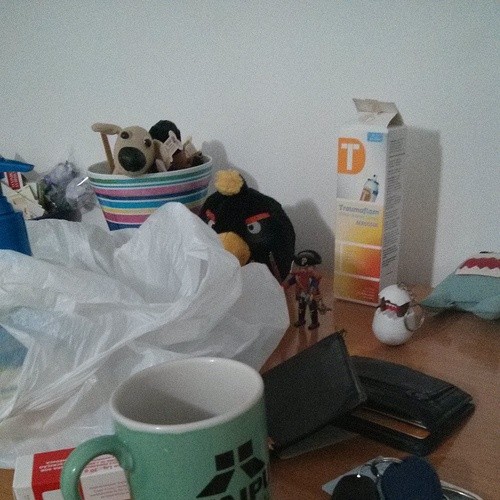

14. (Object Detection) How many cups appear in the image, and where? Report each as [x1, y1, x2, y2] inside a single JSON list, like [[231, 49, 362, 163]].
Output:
[[86, 151, 214, 231], [61, 356, 270, 500]]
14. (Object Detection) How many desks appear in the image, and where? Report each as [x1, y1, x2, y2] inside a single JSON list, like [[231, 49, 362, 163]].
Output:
[[0, 268, 500, 500]]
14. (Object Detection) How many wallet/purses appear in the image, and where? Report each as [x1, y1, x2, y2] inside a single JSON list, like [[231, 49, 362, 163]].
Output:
[[255, 330, 367, 461], [328, 353, 477, 454]]
